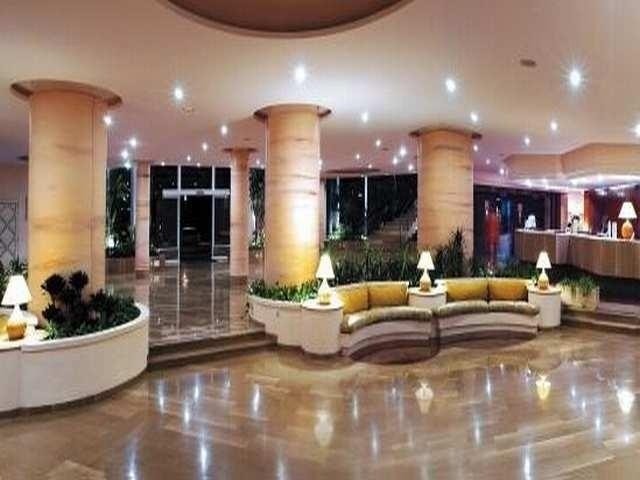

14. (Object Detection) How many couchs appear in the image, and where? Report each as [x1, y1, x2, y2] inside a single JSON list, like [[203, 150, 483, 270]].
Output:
[[332, 277, 540, 357]]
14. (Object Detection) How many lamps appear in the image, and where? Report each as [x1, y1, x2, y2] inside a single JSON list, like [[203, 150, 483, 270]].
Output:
[[618, 201, 637, 238]]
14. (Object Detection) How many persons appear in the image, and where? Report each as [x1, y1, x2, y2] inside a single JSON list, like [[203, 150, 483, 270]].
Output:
[[511, 201, 527, 231], [483, 204, 503, 262]]
